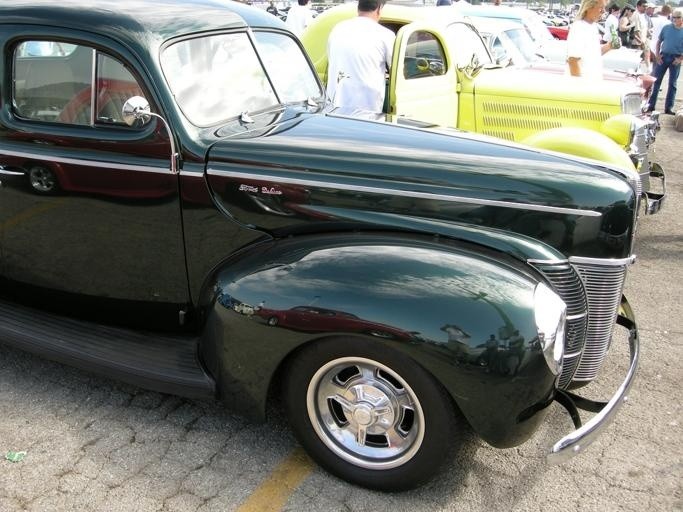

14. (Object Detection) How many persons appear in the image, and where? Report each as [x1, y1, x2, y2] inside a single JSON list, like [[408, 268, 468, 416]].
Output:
[[287, 0, 315, 38], [603, 0, 683, 114], [566, 0, 621, 76], [267, 1, 278, 16], [327, 0, 395, 114]]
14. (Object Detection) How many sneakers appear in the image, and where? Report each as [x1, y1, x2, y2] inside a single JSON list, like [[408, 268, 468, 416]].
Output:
[[665, 109, 675, 115], [648, 107, 655, 111]]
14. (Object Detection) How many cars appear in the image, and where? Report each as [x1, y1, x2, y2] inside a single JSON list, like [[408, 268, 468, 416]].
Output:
[[261, 2, 660, 149], [0, 1, 643, 494], [296, 3, 667, 219]]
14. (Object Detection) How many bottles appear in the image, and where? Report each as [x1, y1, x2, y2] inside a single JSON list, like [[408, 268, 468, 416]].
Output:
[[610, 25, 621, 49]]
